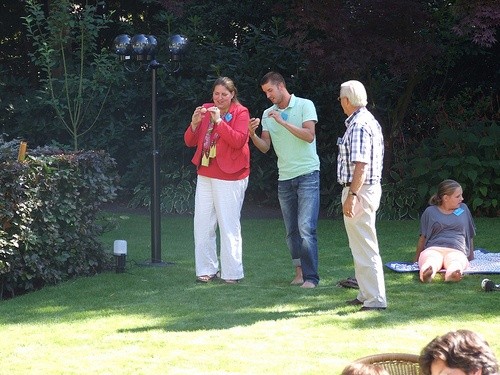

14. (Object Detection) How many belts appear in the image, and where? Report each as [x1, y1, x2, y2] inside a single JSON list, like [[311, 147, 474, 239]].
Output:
[[341, 182, 351, 188]]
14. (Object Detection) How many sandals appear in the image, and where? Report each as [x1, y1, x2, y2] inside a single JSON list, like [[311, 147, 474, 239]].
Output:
[[195, 270, 240, 284]]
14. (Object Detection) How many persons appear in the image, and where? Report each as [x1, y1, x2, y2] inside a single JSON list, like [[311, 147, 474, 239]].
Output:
[[247, 72, 321, 288], [336, 79, 387, 311], [418, 329, 500, 375], [341, 363, 389, 375], [414, 180, 477, 282], [184, 77, 252, 283]]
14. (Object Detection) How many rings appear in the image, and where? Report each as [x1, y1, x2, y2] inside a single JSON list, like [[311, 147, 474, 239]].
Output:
[[214, 107, 217, 110], [346, 211, 349, 213]]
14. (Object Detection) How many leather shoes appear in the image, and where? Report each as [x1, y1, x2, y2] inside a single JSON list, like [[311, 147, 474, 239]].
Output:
[[346, 298, 387, 311]]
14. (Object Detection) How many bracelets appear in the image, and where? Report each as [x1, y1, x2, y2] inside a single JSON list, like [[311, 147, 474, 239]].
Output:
[[348, 190, 358, 196], [216, 118, 221, 124]]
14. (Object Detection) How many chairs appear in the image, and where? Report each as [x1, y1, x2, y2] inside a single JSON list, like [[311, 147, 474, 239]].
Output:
[[341, 352, 424, 375]]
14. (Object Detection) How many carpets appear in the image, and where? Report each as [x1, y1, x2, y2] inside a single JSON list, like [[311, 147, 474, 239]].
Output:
[[385, 249, 500, 275]]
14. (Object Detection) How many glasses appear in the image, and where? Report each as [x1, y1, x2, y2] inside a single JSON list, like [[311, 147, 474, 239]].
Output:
[[338, 96, 345, 101]]
